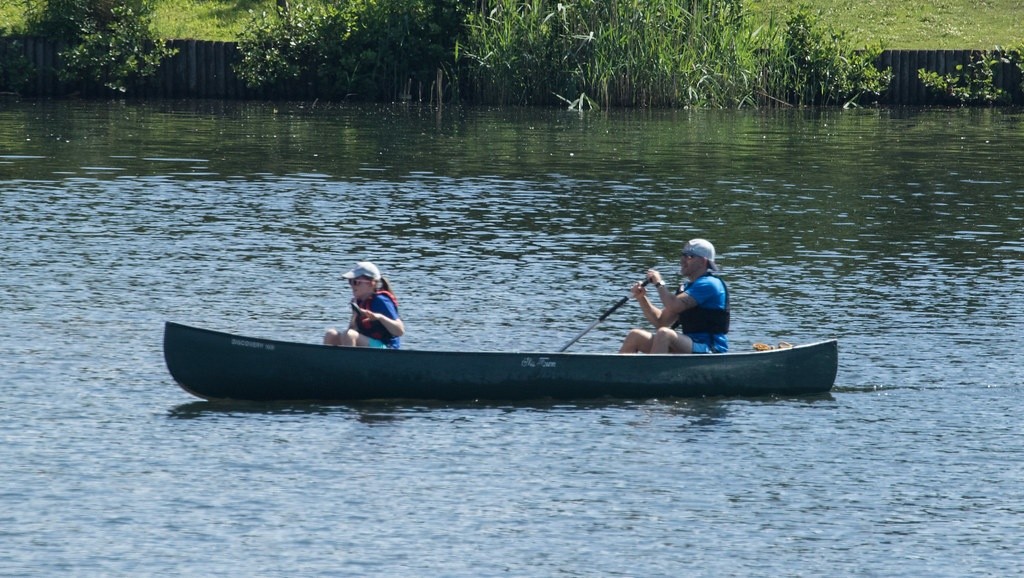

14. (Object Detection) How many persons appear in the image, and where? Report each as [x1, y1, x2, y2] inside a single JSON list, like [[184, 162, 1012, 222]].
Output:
[[618, 238, 730, 354], [323, 261, 405, 350]]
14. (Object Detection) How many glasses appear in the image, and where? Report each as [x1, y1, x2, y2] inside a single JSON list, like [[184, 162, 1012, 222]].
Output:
[[348, 279, 371, 286], [683, 254, 703, 261]]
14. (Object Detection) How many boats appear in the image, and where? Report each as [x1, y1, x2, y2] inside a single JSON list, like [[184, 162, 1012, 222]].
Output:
[[164, 319, 839, 402]]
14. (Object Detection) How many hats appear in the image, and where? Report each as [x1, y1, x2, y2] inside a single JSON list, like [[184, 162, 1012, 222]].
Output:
[[341, 262, 381, 281], [682, 238, 719, 271]]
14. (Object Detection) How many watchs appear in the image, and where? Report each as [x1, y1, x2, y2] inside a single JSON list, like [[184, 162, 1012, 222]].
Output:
[[655, 280, 665, 288]]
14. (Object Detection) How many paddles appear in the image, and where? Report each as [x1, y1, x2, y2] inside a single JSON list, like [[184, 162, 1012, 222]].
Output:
[[552, 271, 663, 357], [336, 299, 369, 342]]
